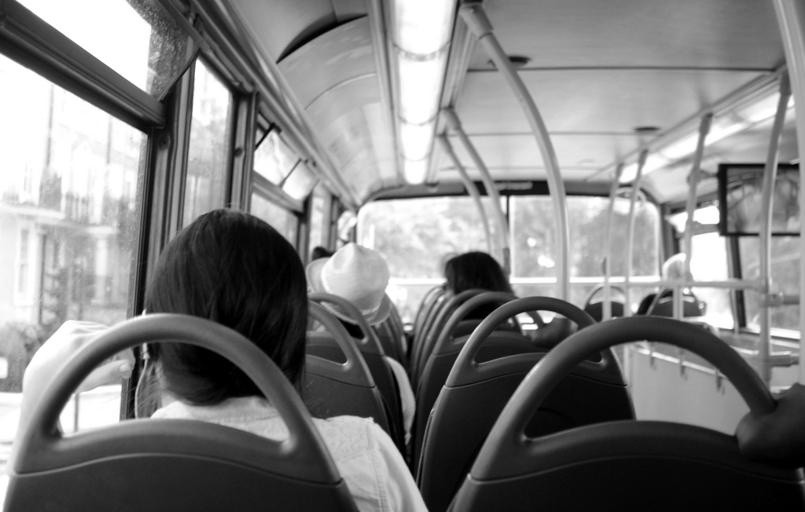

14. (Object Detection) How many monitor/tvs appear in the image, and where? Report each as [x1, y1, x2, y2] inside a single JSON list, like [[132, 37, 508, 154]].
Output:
[[719, 163, 800, 237]]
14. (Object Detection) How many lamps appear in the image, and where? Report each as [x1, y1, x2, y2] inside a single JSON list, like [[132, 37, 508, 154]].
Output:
[[378, 1, 458, 188], [614, 65, 797, 185]]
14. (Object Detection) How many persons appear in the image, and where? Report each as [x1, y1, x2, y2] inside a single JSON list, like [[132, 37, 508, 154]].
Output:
[[636, 253, 686, 316], [443, 252, 514, 321], [300, 242, 417, 445], [21, 206, 435, 512]]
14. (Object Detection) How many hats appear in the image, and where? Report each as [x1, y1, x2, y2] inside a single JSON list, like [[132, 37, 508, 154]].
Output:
[[304, 243, 393, 326]]
